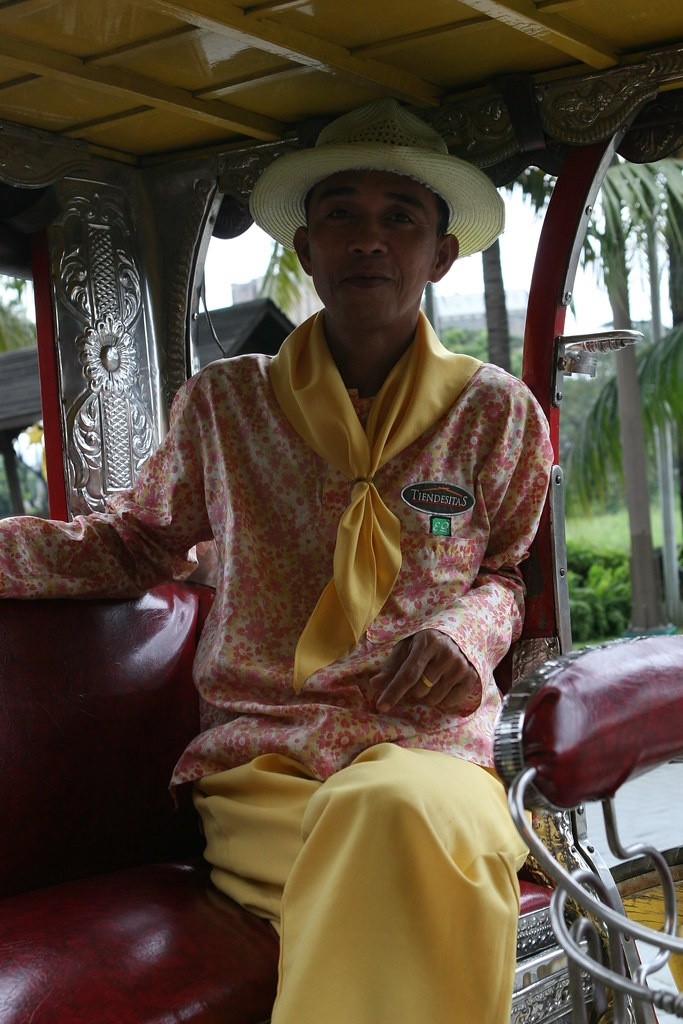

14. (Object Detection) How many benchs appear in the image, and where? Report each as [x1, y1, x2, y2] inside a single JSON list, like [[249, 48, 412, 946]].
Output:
[[1, 582, 602, 1024]]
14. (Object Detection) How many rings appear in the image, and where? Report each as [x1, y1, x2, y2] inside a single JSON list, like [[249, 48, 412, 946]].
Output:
[[421, 674, 433, 688]]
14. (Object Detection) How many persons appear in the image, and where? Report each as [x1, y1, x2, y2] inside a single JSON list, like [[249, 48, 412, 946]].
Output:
[[0, 98, 557, 1024]]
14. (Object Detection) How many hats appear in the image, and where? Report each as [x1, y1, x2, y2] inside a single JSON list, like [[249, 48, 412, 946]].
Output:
[[249, 97, 505, 260]]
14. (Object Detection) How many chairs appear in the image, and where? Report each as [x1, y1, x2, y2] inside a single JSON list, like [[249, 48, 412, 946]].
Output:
[[509, 633, 683, 1024]]
